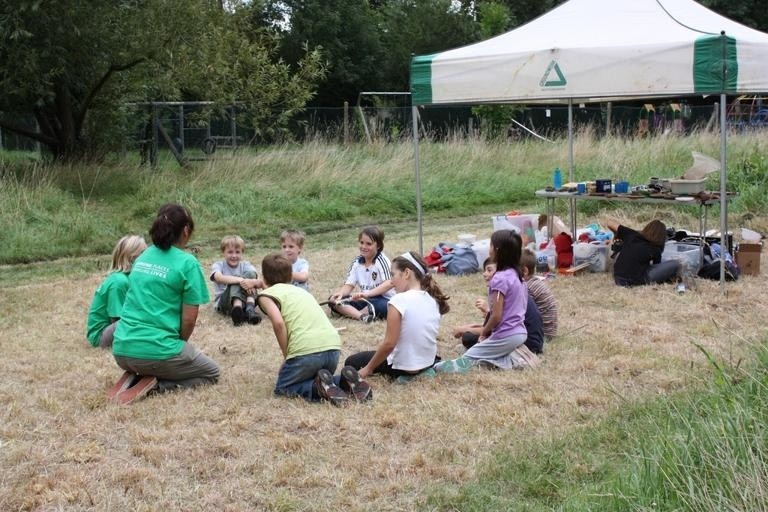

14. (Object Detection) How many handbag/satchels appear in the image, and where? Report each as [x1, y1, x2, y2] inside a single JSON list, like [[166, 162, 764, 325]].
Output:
[[437, 240, 480, 276]]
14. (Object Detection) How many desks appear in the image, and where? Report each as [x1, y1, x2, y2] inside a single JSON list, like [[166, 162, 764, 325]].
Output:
[[535, 180, 740, 273]]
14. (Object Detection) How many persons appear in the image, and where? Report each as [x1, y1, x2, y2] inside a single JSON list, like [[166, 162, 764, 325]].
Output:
[[462, 230, 542, 371], [519, 249, 558, 344], [209, 234, 262, 326], [87, 236, 147, 346], [109, 204, 219, 407], [328, 225, 396, 324], [344, 251, 471, 384], [280, 229, 308, 292], [534, 214, 548, 249], [453, 257, 544, 355], [258, 253, 373, 408], [607, 220, 682, 286]]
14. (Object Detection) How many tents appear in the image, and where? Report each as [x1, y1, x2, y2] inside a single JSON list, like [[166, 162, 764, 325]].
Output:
[[410, 0, 768, 294]]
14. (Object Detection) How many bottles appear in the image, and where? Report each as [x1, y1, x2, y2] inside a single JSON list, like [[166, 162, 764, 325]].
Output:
[[678, 278, 685, 296], [554, 167, 561, 191]]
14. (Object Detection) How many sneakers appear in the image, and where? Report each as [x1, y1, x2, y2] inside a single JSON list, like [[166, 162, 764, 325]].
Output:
[[510, 348, 536, 369], [434, 357, 469, 372], [396, 368, 436, 384], [246, 310, 262, 323], [116, 374, 158, 405], [520, 344, 538, 360], [313, 369, 347, 408], [362, 314, 377, 323], [106, 370, 135, 399], [340, 365, 374, 403], [230, 304, 244, 325]]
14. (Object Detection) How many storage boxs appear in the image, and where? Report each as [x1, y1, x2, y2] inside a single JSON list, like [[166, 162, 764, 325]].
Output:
[[492, 212, 541, 246], [572, 240, 610, 275], [735, 241, 762, 277], [659, 242, 704, 277]]
